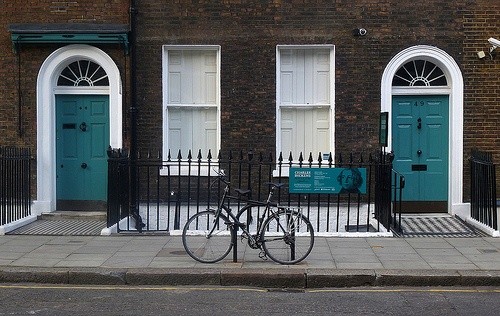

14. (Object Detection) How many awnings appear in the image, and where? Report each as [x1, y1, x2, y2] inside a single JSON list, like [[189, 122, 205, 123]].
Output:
[[11, 24, 128, 44]]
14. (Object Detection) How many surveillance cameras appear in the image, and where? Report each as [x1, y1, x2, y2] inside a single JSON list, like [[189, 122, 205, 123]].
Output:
[[488, 37, 500, 48]]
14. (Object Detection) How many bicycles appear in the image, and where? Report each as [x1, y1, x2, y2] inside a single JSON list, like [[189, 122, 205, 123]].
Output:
[[182, 169, 314, 264]]
